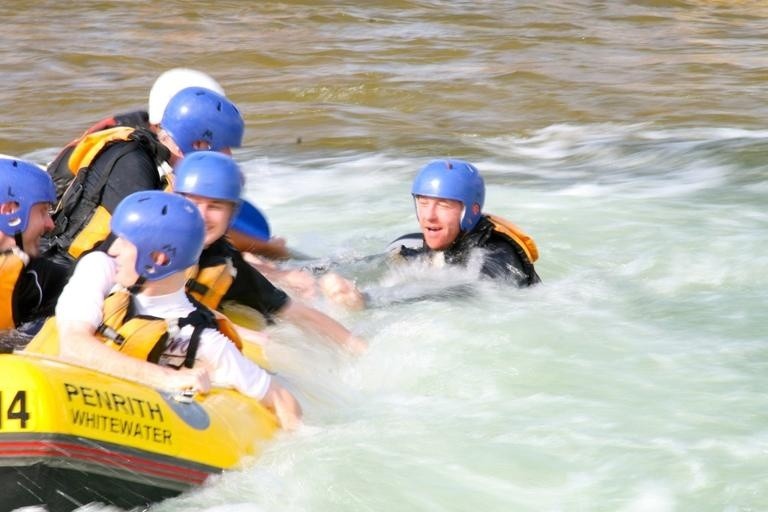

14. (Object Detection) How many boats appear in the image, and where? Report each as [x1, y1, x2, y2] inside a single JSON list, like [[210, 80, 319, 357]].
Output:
[[0, 327, 305, 512]]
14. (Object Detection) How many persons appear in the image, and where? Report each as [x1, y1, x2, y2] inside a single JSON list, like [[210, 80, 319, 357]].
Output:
[[38, 68, 363, 430], [320, 160, 540, 310], [0, 157, 65, 352]]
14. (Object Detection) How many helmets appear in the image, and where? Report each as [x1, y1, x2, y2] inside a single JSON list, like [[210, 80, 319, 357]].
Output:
[[149, 68, 226, 125], [160, 87, 244, 156], [0, 154, 58, 236], [172, 151, 243, 201], [111, 190, 205, 281], [412, 160, 485, 231]]
